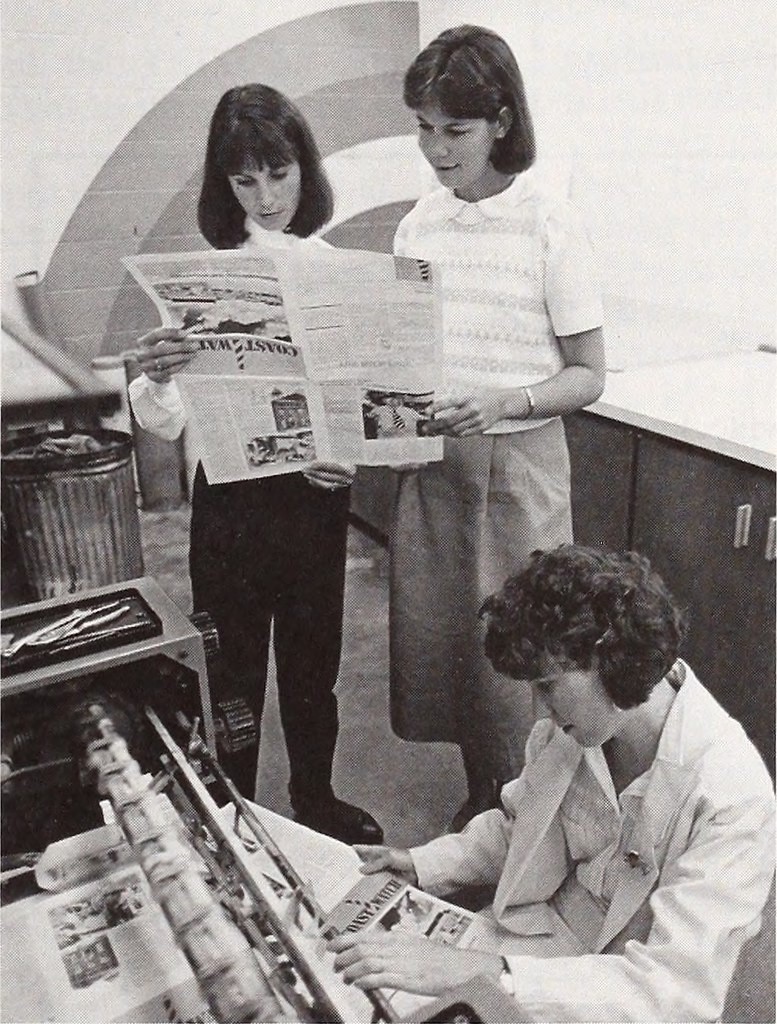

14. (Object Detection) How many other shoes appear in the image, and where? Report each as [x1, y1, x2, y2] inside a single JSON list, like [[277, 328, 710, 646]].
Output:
[[295, 798, 384, 845], [448, 778, 509, 833]]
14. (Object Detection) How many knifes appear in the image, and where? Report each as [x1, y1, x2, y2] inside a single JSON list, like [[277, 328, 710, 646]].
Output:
[[2, 601, 147, 657]]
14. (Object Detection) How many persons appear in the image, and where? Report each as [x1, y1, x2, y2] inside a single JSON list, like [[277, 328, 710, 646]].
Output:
[[389, 24, 606, 911], [60, 889, 136, 980], [184, 305, 309, 466], [124, 82, 386, 850], [365, 395, 430, 442], [323, 544, 777, 1024]]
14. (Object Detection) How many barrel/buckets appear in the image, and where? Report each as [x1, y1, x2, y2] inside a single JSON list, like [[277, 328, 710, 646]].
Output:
[[1, 429, 144, 605]]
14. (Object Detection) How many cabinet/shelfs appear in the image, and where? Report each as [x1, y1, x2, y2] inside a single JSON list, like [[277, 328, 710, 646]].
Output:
[[561, 351, 776, 791]]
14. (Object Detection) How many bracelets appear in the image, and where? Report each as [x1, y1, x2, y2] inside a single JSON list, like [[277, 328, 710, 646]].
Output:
[[499, 955, 515, 998], [523, 386, 536, 418]]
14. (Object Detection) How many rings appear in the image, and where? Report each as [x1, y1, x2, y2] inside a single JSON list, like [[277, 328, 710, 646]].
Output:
[[332, 482, 339, 490], [461, 422, 472, 429], [153, 358, 159, 371]]
